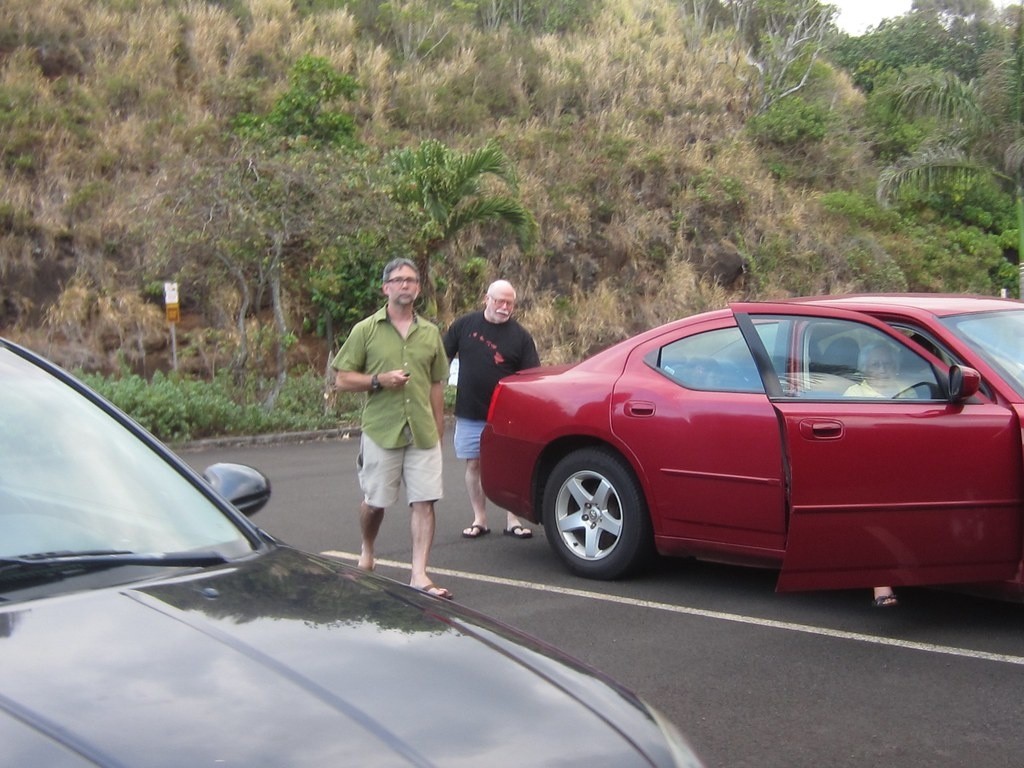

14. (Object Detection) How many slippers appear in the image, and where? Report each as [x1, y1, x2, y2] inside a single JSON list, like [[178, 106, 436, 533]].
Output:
[[503, 525, 533, 537], [462, 525, 491, 539], [421, 584, 453, 598]]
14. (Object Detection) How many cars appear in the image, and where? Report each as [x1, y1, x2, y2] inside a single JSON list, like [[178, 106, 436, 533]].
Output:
[[1, 336, 709, 768], [477, 292, 1023, 593]]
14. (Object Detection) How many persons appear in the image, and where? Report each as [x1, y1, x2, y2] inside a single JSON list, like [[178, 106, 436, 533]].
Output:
[[330, 259, 451, 599], [843, 341, 919, 608], [441, 280, 542, 538]]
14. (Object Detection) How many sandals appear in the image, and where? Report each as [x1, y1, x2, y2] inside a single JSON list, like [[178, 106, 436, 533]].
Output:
[[874, 594, 898, 606]]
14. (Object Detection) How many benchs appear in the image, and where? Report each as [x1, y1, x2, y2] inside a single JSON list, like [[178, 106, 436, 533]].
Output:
[[660, 358, 744, 387]]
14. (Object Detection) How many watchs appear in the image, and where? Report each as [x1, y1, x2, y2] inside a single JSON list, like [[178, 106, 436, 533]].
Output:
[[372, 375, 382, 391]]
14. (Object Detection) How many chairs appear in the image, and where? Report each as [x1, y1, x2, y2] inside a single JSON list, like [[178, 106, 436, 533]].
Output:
[[815, 338, 860, 374]]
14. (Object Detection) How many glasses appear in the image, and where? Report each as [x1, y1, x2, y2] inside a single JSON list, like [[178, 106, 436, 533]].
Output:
[[867, 362, 893, 368], [386, 276, 419, 284], [489, 295, 515, 307]]
